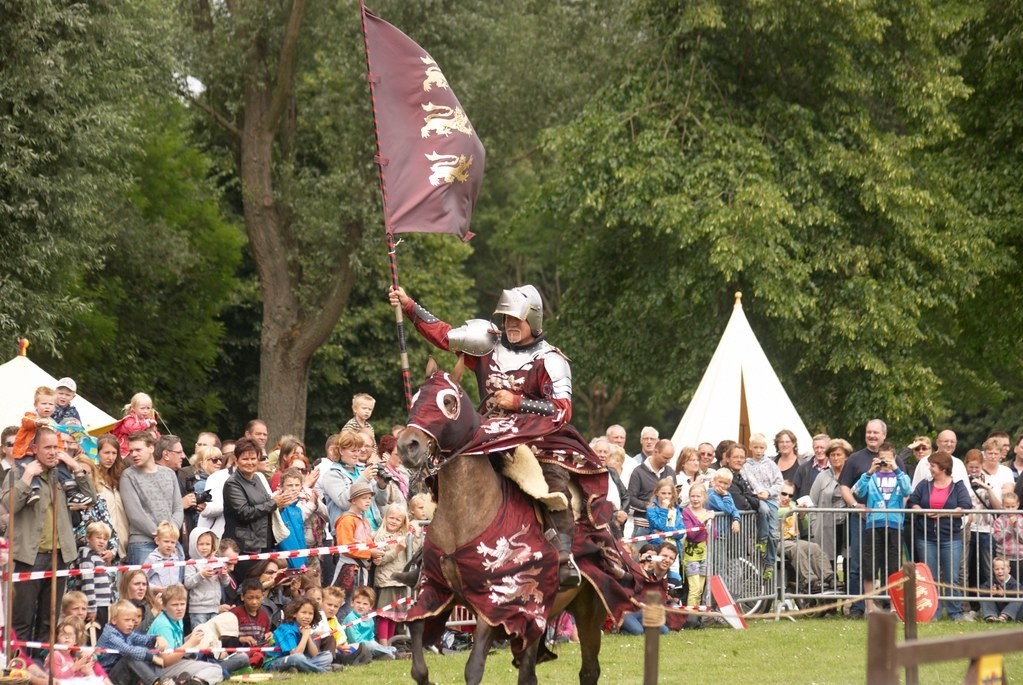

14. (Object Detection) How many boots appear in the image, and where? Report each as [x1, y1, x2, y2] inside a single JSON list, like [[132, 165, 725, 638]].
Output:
[[552, 533, 579, 586]]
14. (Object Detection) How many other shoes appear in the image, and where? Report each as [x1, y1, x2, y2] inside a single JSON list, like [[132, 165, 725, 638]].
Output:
[[985, 615, 1007, 622], [955, 611, 978, 623], [157, 671, 200, 685], [826, 574, 844, 590], [342, 641, 372, 668], [759, 540, 767, 553], [970, 609, 980, 617], [806, 580, 828, 592], [763, 566, 773, 581], [846, 606, 864, 621]]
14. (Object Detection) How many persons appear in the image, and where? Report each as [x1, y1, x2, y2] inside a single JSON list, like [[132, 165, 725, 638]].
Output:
[[389, 284, 579, 592], [0, 377, 427, 685], [591, 419, 1023, 635]]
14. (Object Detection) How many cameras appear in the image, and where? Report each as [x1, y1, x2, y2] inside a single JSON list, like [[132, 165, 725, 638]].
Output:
[[376, 463, 392, 484], [878, 458, 887, 466], [185, 475, 213, 513], [966, 473, 985, 490], [645, 554, 663, 563]]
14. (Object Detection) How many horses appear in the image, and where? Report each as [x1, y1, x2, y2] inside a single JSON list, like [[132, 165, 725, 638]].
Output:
[[395, 353, 612, 685]]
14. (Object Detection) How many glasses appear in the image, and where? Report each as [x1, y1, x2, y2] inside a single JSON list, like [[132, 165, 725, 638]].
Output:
[[780, 492, 794, 499], [166, 449, 183, 455], [914, 446, 928, 451], [2, 441, 14, 447], [700, 452, 713, 458], [207, 457, 223, 464], [298, 468, 308, 475], [264, 569, 276, 575]]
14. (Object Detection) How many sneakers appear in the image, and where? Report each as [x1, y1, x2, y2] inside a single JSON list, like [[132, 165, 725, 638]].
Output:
[[67, 491, 93, 506], [27, 490, 40, 506]]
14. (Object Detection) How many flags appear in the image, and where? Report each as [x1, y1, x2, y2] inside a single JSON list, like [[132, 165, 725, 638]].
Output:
[[364, 6, 486, 243]]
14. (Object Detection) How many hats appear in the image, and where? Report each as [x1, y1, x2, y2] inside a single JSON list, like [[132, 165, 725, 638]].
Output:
[[348, 482, 376, 504], [57, 377, 77, 393]]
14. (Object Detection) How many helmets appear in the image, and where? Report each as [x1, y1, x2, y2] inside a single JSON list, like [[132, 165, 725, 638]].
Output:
[[493, 285, 544, 337]]
